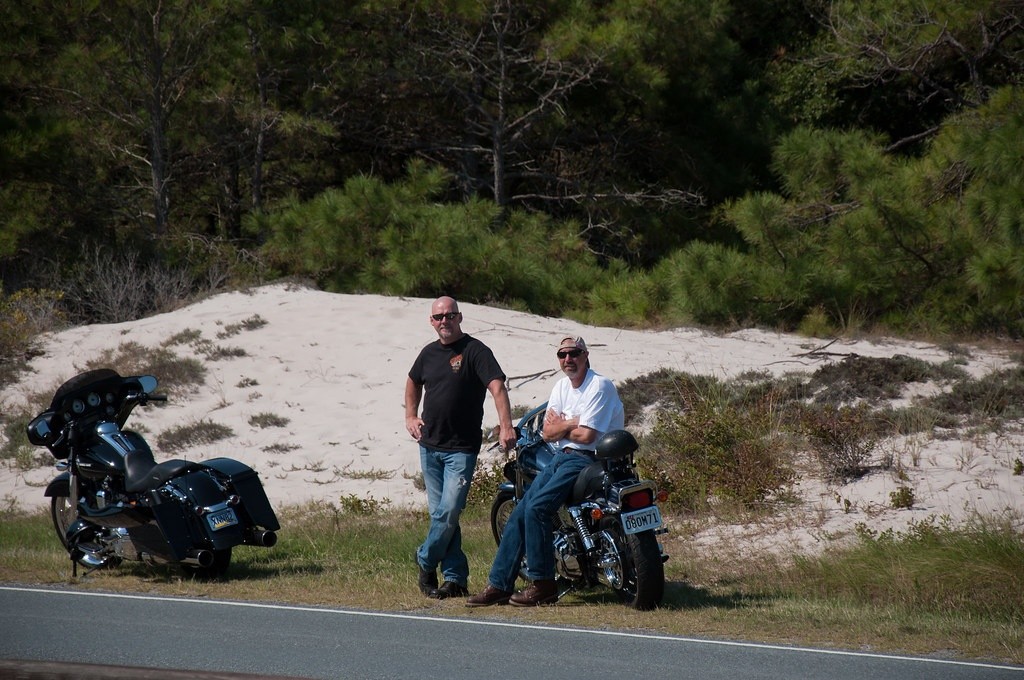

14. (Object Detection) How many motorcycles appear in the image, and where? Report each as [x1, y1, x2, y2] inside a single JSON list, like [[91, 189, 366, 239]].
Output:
[[489, 401, 670, 610], [26, 368, 281, 581]]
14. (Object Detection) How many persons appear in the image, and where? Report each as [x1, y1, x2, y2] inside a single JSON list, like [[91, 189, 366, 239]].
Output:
[[464, 335, 625, 606], [404, 296, 517, 600]]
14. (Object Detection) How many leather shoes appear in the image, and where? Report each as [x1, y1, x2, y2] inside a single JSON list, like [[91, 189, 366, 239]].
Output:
[[465, 585, 514, 607], [414, 550, 439, 598], [429, 581, 468, 600], [509, 579, 559, 607]]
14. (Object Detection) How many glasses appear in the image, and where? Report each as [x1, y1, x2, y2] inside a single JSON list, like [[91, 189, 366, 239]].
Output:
[[557, 349, 583, 358], [432, 312, 459, 321]]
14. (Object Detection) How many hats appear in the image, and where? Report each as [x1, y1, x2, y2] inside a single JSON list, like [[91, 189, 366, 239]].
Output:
[[556, 336, 590, 368]]
